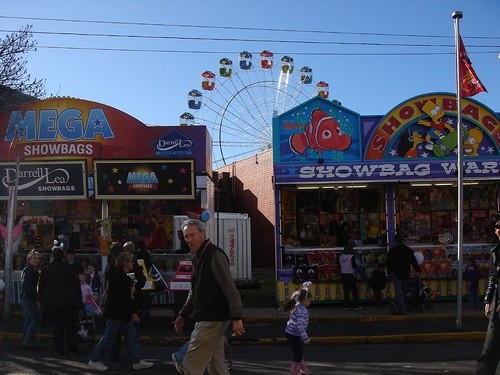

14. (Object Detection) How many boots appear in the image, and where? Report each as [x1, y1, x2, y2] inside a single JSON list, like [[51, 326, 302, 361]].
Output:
[[300, 357, 313, 375], [290, 362, 300, 375]]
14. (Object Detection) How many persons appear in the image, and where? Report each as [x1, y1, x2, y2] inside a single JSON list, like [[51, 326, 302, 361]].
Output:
[[465, 258, 481, 310], [19, 249, 41, 350], [281, 281, 314, 375], [61, 240, 157, 359], [87, 251, 154, 372], [338, 243, 361, 312], [366, 262, 387, 311], [337, 220, 354, 246], [38, 246, 84, 357], [387, 234, 421, 315], [475, 216, 500, 375], [172, 219, 246, 375]]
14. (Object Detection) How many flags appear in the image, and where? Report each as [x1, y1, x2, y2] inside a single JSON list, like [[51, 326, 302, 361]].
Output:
[[455, 17, 489, 99]]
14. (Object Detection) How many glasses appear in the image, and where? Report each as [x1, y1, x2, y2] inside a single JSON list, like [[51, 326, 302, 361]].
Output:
[[493, 225, 500, 229]]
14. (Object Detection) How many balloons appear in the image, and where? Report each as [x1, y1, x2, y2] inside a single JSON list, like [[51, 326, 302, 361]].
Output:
[[413, 252, 425, 268]]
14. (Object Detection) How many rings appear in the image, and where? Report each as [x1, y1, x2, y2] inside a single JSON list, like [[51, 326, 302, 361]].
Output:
[[240, 330, 243, 333]]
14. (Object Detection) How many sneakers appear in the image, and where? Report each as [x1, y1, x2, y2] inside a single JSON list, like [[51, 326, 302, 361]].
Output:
[[172, 353, 185, 374], [133, 360, 153, 370], [88, 360, 108, 371]]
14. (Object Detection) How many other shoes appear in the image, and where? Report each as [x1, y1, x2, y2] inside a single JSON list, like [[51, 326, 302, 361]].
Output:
[[344, 303, 406, 318], [23, 324, 91, 356]]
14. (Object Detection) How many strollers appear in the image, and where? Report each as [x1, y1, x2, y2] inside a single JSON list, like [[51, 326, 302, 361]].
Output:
[[390, 271, 432, 314]]
